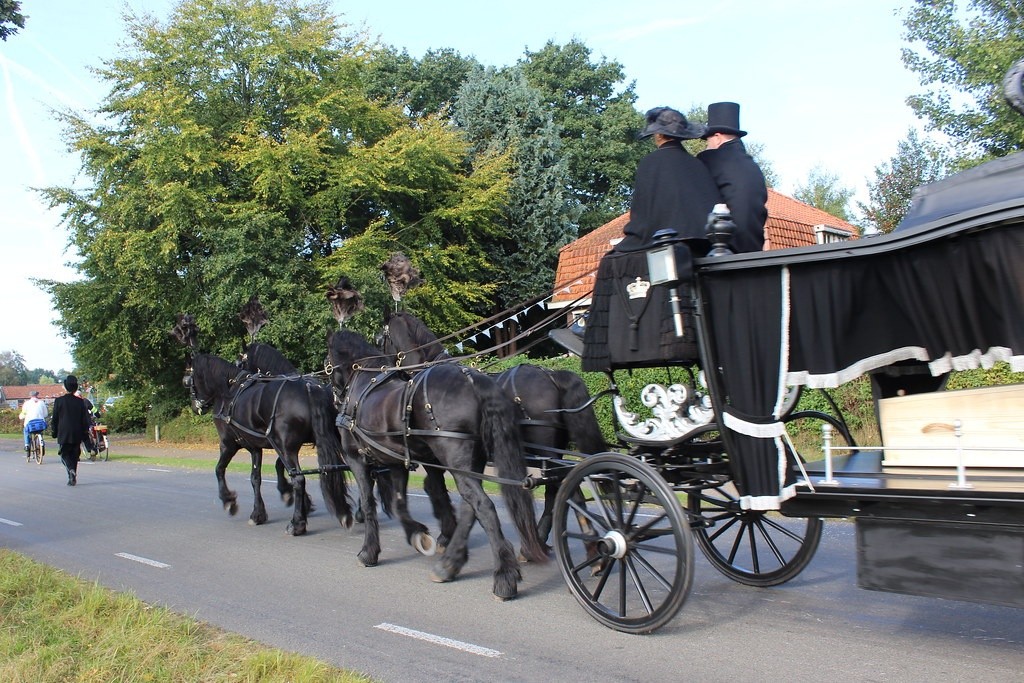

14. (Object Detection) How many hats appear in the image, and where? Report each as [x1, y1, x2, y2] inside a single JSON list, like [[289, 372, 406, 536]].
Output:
[[637, 107, 710, 139], [30, 390, 39, 396], [64, 375, 78, 392], [700, 101, 747, 140]]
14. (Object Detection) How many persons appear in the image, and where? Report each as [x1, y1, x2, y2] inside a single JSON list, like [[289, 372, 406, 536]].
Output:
[[52, 375, 101, 486], [572, 107, 725, 341], [696, 103, 767, 254], [19, 391, 48, 455]]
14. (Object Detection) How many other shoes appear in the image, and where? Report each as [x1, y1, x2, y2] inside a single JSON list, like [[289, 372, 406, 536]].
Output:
[[91, 449, 96, 461], [572, 323, 587, 337], [67, 469, 76, 486], [24, 446, 29, 451]]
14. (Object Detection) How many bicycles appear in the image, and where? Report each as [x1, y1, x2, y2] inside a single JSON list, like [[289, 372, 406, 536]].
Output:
[[23, 422, 47, 465], [78, 410, 109, 462]]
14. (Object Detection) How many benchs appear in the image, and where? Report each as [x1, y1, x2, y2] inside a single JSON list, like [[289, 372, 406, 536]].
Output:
[[547, 237, 715, 371]]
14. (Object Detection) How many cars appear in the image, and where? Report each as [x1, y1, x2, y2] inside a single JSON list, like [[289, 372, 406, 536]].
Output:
[[104, 395, 152, 415]]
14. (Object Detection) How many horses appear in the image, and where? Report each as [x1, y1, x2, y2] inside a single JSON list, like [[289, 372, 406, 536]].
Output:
[[237, 338, 396, 524], [375, 303, 623, 578], [183, 350, 357, 536], [323, 326, 554, 602]]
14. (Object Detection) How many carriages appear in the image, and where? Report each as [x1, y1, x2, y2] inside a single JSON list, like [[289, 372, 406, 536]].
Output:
[[190, 148, 1024, 638]]
